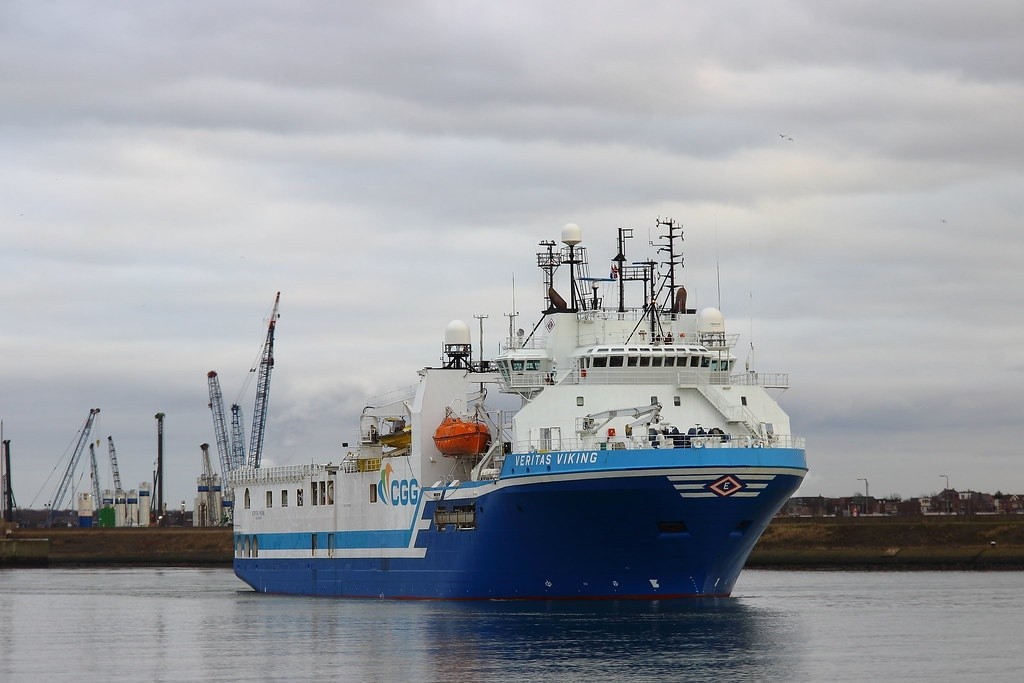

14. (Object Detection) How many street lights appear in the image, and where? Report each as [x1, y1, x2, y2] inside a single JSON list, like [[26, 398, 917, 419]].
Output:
[[940, 475, 949, 513]]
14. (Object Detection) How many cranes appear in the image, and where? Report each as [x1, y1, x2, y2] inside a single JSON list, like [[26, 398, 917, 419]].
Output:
[[21, 289, 280, 527]]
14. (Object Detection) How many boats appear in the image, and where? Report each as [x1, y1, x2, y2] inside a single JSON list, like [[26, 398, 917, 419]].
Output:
[[226, 217, 808, 612], [432, 417, 493, 461]]
